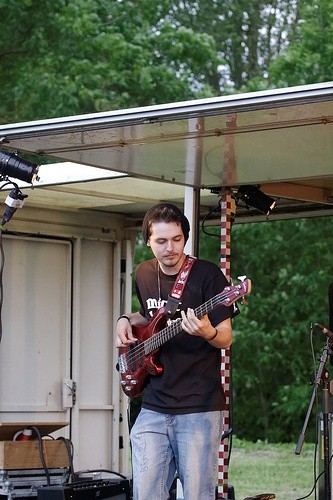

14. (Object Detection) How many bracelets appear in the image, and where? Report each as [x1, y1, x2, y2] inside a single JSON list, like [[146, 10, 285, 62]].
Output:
[[207, 329, 218, 342], [117, 316, 131, 324]]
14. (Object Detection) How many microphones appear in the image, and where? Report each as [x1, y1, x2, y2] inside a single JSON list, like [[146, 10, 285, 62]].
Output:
[[314, 323, 333, 338]]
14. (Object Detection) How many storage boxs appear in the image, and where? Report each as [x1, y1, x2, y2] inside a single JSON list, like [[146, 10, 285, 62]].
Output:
[[0, 422, 72, 469]]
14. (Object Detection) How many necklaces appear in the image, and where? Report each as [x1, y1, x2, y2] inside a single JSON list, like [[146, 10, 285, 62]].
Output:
[[156, 262, 161, 312]]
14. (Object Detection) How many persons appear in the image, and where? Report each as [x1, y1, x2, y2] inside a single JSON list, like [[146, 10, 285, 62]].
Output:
[[115, 202, 240, 500]]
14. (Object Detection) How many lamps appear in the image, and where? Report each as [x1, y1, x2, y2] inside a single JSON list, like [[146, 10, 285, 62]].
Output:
[[0, 150, 41, 227]]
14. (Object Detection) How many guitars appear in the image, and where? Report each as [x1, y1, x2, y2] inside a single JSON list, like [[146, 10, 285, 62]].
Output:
[[115, 276, 251, 397]]
